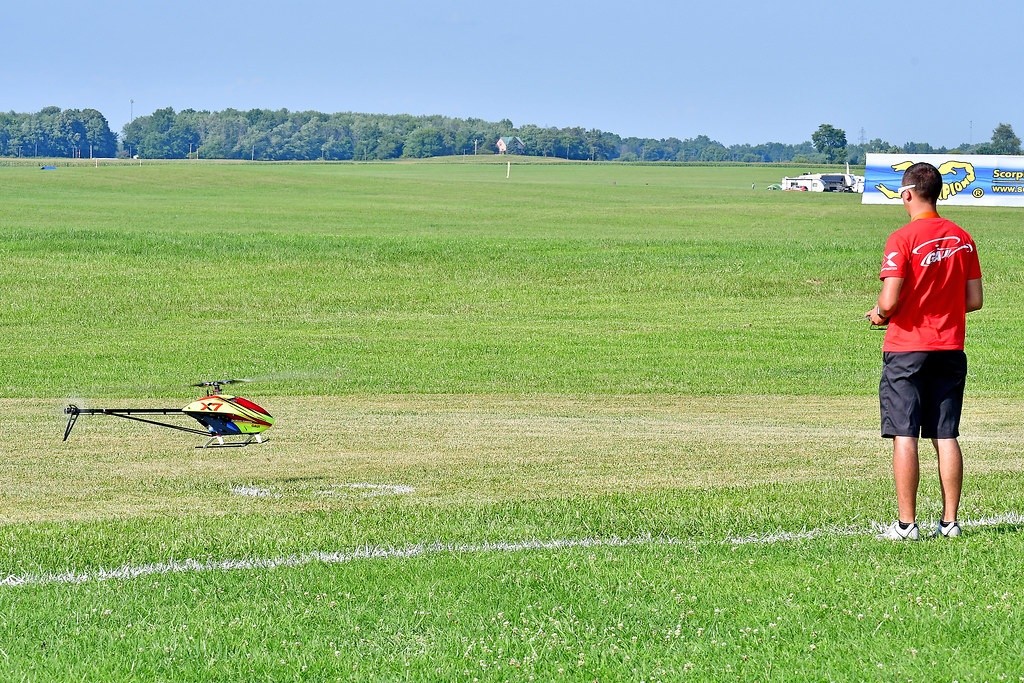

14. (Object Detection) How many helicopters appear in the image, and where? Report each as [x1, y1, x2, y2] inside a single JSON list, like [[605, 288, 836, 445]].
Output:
[[63, 379, 276, 447]]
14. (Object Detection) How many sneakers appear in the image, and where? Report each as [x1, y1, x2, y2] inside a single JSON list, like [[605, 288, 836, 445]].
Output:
[[922, 521, 962, 538], [875, 520, 919, 541]]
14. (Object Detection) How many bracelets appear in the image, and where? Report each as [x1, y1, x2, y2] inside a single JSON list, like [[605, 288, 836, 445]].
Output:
[[877, 306, 884, 320]]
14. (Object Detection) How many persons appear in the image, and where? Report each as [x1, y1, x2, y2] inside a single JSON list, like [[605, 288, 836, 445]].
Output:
[[865, 163, 983, 540]]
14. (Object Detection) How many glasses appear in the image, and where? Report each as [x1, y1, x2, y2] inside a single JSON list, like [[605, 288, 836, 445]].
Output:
[[898, 185, 916, 198]]
